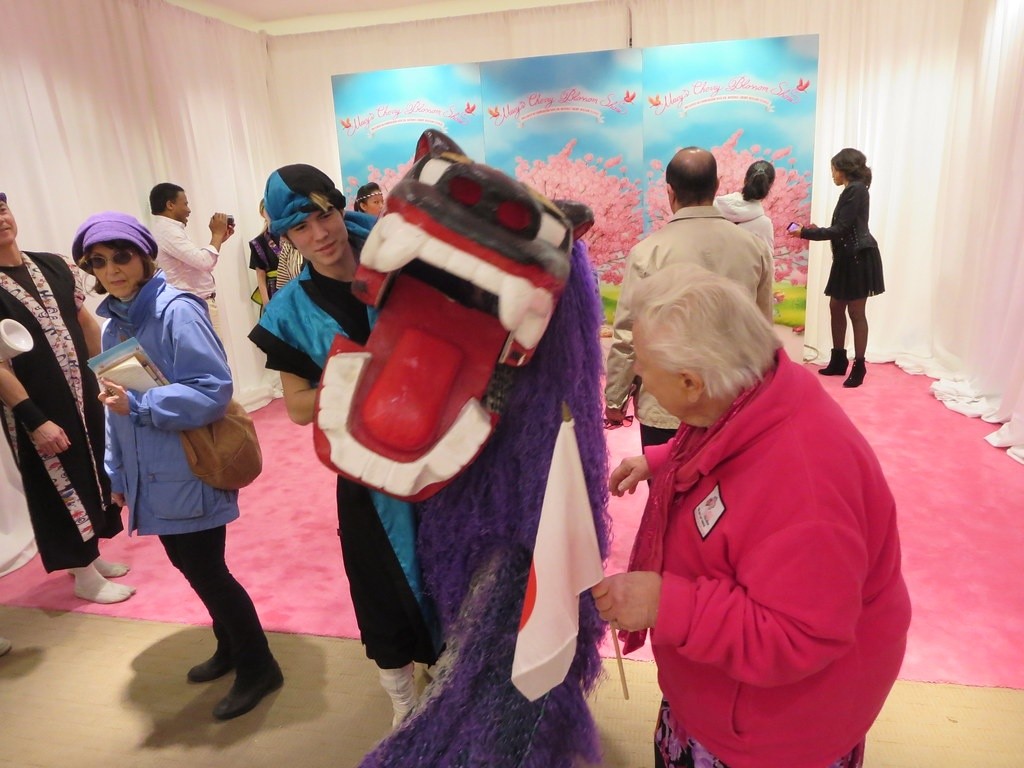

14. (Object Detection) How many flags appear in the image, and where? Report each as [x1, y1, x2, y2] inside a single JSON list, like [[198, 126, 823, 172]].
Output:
[[511, 419, 604, 703]]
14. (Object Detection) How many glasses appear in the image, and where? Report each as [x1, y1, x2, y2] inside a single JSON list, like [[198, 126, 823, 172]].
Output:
[[604, 415, 634, 430], [88, 249, 134, 269]]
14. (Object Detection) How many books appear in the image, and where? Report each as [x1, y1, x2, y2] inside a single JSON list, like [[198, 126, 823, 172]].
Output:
[[99, 354, 160, 396]]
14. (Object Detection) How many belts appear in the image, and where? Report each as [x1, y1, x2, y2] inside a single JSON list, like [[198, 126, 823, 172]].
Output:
[[208, 293, 216, 298]]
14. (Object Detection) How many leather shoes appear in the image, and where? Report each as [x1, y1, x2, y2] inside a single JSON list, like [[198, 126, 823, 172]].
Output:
[[188, 650, 243, 682], [213, 672, 285, 719]]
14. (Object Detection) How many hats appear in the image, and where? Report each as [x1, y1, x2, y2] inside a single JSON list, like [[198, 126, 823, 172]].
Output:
[[72, 211, 159, 276], [264, 165, 347, 237]]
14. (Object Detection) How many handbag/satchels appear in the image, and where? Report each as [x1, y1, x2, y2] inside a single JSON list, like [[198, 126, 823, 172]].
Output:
[[178, 397, 263, 489]]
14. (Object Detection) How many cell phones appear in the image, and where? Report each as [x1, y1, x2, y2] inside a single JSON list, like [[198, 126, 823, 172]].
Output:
[[787, 222, 798, 231]]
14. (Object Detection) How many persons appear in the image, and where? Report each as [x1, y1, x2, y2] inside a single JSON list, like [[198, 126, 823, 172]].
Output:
[[590, 267, 911, 768], [713, 160, 774, 250], [788, 148, 885, 388], [72, 213, 285, 719], [0, 192, 136, 603], [246, 165, 442, 728], [603, 146, 774, 494], [150, 182, 386, 344]]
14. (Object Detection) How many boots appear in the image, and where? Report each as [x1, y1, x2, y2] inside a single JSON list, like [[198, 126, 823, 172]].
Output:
[[819, 349, 849, 376], [843, 358, 866, 387], [380, 660, 418, 732]]
